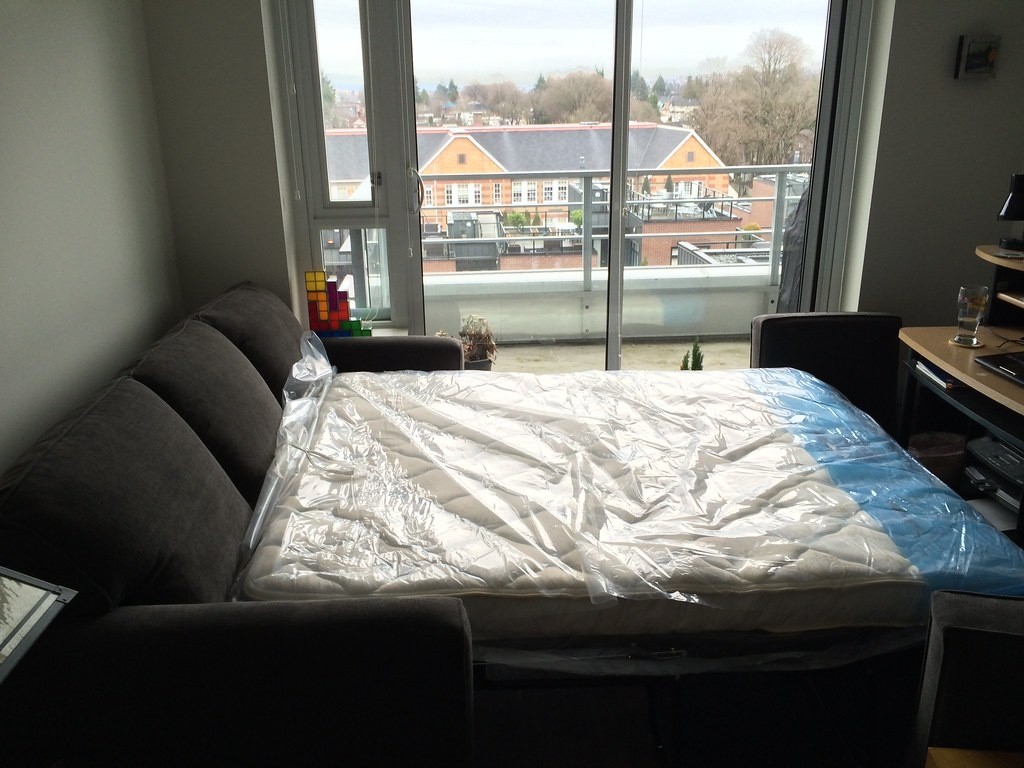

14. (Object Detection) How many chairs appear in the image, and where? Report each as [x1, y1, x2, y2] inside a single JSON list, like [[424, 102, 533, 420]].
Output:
[[650, 190, 718, 218], [900, 591, 1024, 768]]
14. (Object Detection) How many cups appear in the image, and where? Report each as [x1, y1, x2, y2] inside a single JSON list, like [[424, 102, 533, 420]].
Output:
[[954, 284, 988, 345]]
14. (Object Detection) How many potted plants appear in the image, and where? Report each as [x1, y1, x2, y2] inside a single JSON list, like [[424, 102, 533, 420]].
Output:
[[435, 313, 499, 372], [506, 210, 584, 253]]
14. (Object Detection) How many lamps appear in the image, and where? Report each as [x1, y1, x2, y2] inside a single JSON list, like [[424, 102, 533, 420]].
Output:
[[997, 174, 1024, 221]]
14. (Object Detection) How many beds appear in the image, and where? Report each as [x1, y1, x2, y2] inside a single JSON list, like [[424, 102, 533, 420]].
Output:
[[0, 282, 1024, 768]]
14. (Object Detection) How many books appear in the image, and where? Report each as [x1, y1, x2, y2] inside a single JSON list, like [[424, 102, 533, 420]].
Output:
[[916, 360, 971, 389]]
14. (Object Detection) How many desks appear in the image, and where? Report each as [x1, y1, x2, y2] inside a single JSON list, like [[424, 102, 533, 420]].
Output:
[[529, 228, 538, 235], [894, 244, 1024, 550], [549, 227, 558, 236]]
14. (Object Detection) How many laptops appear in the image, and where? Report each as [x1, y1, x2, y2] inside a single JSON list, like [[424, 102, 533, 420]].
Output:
[[974, 351, 1024, 386]]
